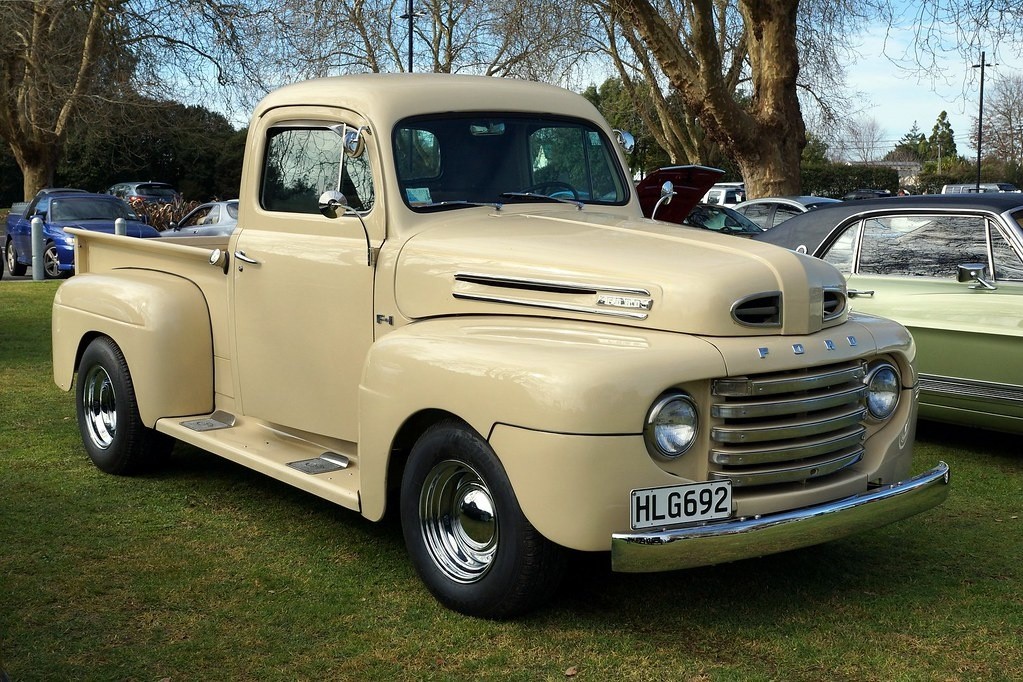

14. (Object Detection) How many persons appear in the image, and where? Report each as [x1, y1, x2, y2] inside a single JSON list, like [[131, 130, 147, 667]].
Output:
[[148, 200, 204, 232], [897, 190, 905, 196]]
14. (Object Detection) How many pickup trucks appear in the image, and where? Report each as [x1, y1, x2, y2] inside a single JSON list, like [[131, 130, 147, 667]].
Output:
[[4, 187, 161, 279]]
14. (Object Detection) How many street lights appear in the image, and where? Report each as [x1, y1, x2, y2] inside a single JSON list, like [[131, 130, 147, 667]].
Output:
[[970, 62, 999, 192], [395, 10, 426, 73]]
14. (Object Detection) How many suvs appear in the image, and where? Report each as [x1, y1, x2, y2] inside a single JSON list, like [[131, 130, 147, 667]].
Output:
[[159, 200, 241, 236]]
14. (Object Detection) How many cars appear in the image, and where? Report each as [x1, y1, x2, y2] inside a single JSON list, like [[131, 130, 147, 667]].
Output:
[[50, 70, 951, 623], [680, 182, 1022, 442], [105, 181, 181, 220], [547, 190, 620, 203]]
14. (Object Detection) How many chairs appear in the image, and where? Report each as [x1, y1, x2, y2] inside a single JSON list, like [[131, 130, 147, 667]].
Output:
[[435, 142, 527, 206], [52, 207, 79, 221]]
